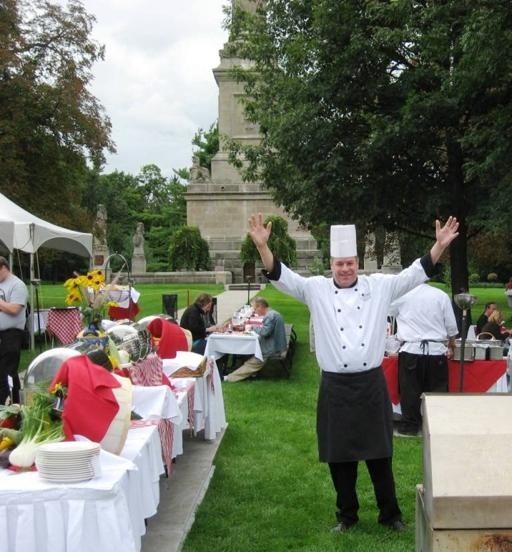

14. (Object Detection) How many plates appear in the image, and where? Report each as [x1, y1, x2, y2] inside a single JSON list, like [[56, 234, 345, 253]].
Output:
[[34, 441, 102, 483]]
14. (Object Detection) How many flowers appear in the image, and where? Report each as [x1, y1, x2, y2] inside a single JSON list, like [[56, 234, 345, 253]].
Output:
[[63, 269, 121, 325]]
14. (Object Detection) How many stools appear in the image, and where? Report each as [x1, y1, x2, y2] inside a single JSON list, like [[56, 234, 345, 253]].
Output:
[[268, 324, 296, 379]]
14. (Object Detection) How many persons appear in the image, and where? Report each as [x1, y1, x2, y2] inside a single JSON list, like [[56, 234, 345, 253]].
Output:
[[218, 295, 287, 383], [476, 302, 497, 335], [386, 280, 460, 436], [178, 293, 229, 380], [482, 311, 512, 360], [244, 211, 460, 533], [0, 255, 29, 406]]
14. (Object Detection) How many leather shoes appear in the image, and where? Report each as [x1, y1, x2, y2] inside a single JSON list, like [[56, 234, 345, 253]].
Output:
[[390, 520, 406, 531], [330, 520, 352, 533]]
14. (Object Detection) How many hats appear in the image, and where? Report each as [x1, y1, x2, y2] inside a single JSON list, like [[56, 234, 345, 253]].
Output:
[[330, 223, 358, 258]]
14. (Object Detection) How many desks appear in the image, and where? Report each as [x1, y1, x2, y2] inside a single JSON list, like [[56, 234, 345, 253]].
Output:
[[382, 357, 507, 420], [0, 307, 266, 551]]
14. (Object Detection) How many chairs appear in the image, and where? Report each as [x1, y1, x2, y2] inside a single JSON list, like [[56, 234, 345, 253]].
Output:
[[162, 295, 177, 320]]
